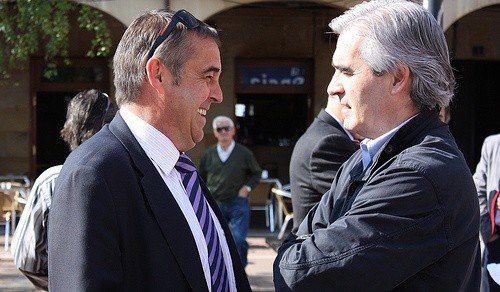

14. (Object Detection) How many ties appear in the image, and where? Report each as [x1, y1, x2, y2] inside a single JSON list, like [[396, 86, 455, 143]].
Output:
[[174, 152, 231, 292]]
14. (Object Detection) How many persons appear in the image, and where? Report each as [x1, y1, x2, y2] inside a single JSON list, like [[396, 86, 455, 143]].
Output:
[[472, 134, 499, 292], [199, 116, 262, 268], [272, 0, 482, 292], [10, 89, 118, 292], [47, 8, 253, 292], [289, 94, 362, 230]]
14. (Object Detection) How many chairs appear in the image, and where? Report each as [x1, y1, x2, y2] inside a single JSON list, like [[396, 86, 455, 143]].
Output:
[[1, 174, 34, 247], [247, 170, 297, 239]]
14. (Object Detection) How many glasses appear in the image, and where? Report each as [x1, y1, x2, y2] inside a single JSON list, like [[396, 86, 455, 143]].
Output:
[[144, 9, 200, 74], [216, 125, 231, 132]]
